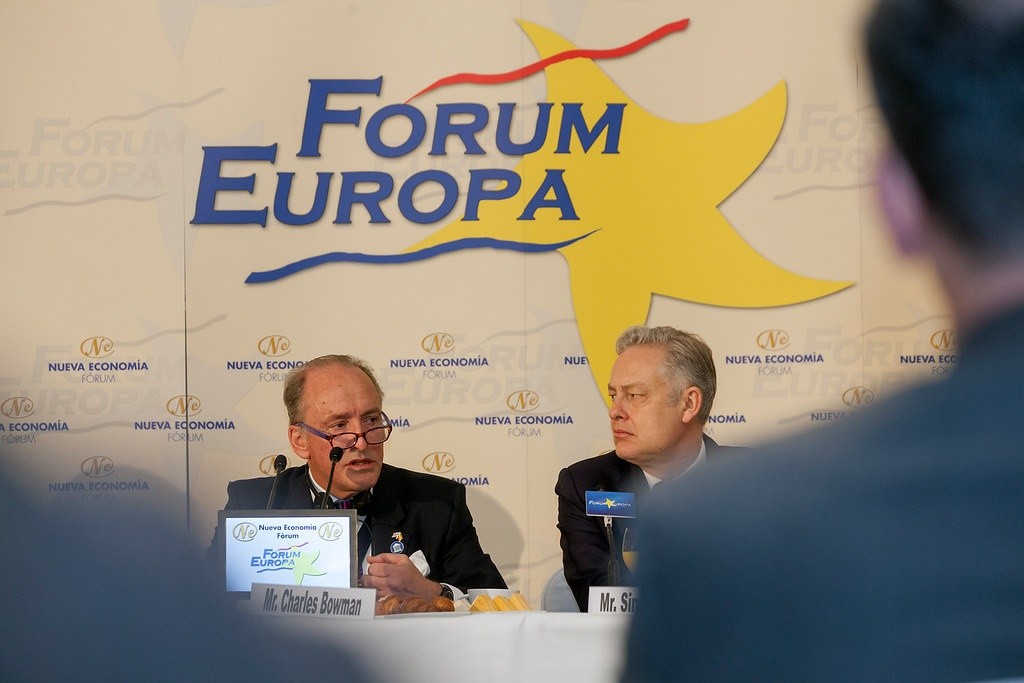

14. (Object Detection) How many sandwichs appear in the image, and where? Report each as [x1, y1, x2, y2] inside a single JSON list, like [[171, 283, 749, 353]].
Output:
[[467, 591, 529, 612]]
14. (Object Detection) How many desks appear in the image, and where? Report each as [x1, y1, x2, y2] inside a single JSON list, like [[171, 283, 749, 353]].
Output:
[[242, 600, 634, 683]]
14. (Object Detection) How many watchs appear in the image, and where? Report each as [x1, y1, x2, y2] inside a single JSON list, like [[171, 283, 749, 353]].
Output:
[[440, 584, 453, 601]]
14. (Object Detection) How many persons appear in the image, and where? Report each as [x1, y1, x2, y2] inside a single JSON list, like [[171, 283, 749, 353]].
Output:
[[615, 1, 1024, 683], [206, 355, 509, 606], [554, 326, 753, 613]]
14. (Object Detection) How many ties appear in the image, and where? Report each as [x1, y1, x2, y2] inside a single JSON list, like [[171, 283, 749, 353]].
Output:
[[333, 498, 354, 509], [651, 480, 684, 514]]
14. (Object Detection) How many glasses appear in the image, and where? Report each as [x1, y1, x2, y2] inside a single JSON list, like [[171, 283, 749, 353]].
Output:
[[292, 410, 393, 450]]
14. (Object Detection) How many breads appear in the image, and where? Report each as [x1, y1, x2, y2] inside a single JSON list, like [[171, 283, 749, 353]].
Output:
[[375, 593, 455, 616]]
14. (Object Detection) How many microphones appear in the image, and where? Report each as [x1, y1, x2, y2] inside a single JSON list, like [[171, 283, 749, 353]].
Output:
[[266, 455, 287, 510], [321, 447, 343, 510], [585, 472, 637, 586]]
